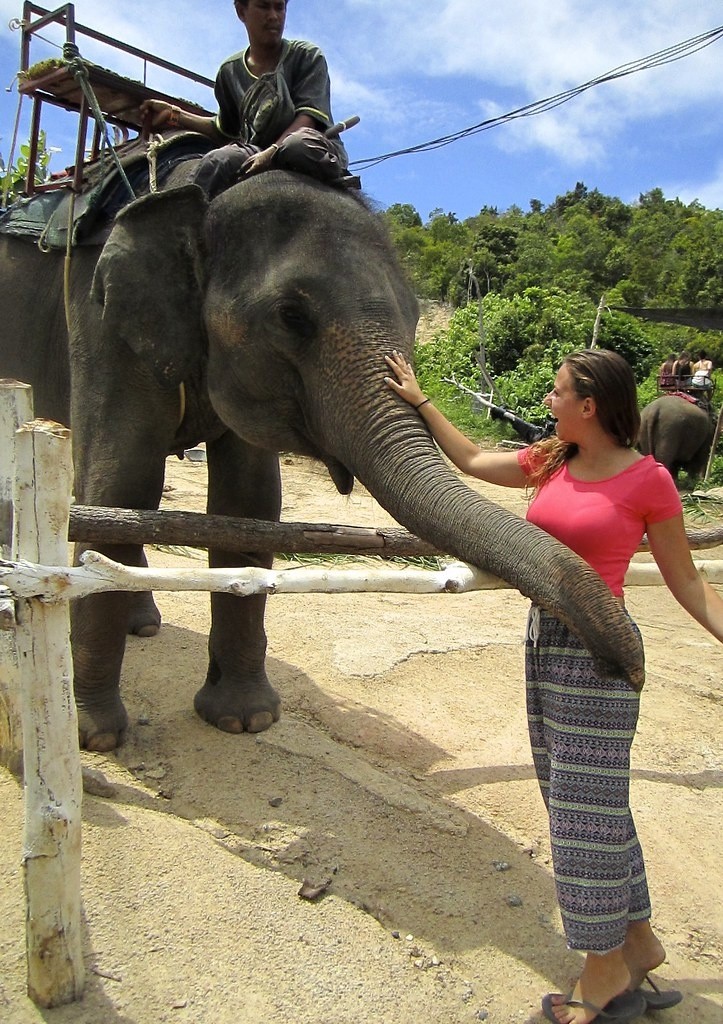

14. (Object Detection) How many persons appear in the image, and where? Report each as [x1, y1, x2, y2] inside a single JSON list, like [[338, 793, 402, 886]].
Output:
[[692, 348, 712, 386], [139, 0, 362, 200], [384, 350, 723, 1024], [672, 351, 695, 386], [660, 353, 678, 387]]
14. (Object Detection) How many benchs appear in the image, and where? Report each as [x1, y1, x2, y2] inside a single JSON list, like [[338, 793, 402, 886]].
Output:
[[656, 375, 715, 401], [13, 1, 217, 194]]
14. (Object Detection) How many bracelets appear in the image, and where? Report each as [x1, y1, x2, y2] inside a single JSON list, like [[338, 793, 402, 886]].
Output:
[[415, 399, 430, 409], [272, 144, 278, 149], [167, 106, 181, 125]]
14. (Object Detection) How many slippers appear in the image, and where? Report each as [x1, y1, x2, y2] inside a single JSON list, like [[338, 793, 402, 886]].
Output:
[[634, 975, 683, 1009], [542, 987, 646, 1024]]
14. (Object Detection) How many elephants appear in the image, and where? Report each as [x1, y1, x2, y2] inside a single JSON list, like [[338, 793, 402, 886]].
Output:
[[639, 395, 716, 490], [2, 134, 642, 748]]
